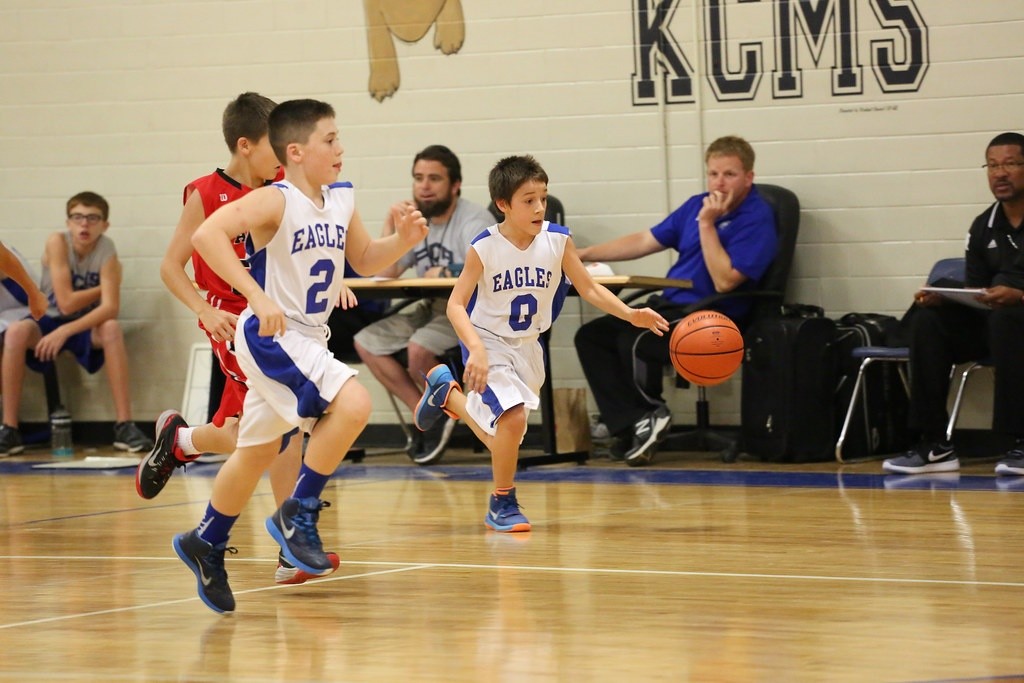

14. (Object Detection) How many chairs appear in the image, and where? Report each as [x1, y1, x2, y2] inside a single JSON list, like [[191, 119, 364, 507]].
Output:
[[620, 185, 802, 462], [342, 196, 563, 464], [835, 258, 1005, 465]]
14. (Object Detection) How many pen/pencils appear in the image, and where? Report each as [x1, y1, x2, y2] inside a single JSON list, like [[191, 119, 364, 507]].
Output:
[[920, 296, 923, 302]]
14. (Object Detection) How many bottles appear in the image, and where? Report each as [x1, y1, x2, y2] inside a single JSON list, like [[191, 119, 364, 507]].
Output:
[[51, 411, 73, 461]]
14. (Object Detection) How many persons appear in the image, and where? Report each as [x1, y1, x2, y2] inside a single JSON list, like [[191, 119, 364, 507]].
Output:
[[133, 91, 359, 585], [170, 100, 431, 611], [883, 132, 1024, 474], [574, 137, 774, 464], [414, 156, 670, 531], [0, 191, 152, 456], [352, 144, 489, 465]]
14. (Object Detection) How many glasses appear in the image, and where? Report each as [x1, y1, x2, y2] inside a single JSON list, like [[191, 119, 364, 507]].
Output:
[[981, 160, 1024, 172], [69, 213, 104, 224]]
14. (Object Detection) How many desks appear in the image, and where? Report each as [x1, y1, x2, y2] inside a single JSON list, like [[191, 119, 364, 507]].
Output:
[[341, 276, 694, 471]]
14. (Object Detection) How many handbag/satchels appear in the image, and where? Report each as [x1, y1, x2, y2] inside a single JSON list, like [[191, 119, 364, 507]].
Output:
[[739, 303, 837, 462], [834, 311, 899, 458]]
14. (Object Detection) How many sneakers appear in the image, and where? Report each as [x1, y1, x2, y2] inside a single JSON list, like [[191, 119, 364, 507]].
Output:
[[413, 363, 462, 432], [883, 441, 959, 471], [414, 417, 459, 464], [0, 425, 24, 456], [266, 496, 334, 575], [484, 487, 531, 532], [113, 422, 153, 453], [994, 448, 1024, 474], [136, 408, 202, 500], [275, 552, 339, 584], [172, 527, 239, 615], [625, 407, 671, 465]]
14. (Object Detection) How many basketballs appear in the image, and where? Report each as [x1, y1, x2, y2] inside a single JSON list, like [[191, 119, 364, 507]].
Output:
[[669, 310, 744, 386]]
